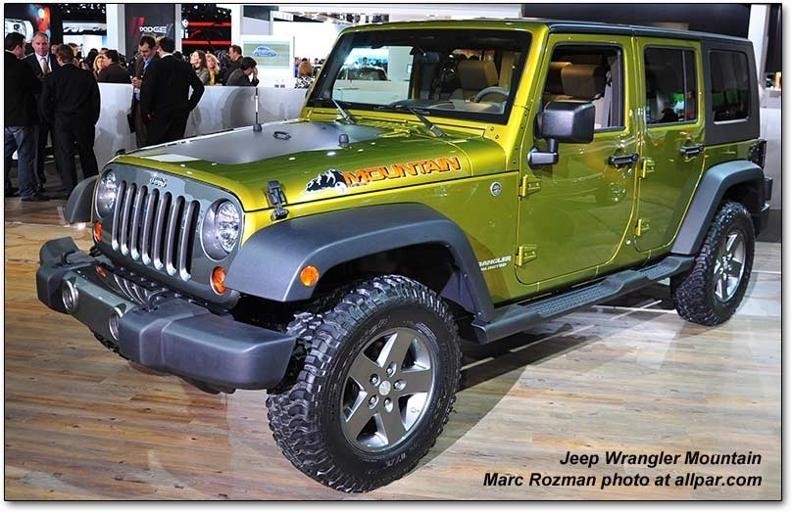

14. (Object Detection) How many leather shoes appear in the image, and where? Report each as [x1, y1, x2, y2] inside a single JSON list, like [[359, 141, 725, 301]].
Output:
[[8, 183, 70, 202]]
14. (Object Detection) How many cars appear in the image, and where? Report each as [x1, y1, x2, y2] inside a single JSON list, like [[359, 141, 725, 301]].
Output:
[[253, 45, 277, 57]]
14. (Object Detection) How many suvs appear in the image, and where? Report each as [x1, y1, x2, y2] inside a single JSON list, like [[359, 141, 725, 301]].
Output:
[[36, 14, 776, 501]]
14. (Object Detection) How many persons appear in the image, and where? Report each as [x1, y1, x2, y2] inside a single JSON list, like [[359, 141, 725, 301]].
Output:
[[4, 31, 388, 201]]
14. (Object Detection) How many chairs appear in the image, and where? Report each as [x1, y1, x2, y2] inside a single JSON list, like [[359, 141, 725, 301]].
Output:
[[446, 58, 506, 103], [543, 60, 613, 129]]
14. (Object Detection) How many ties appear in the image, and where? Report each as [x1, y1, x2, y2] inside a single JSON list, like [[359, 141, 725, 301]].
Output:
[[43, 59, 49, 74]]
